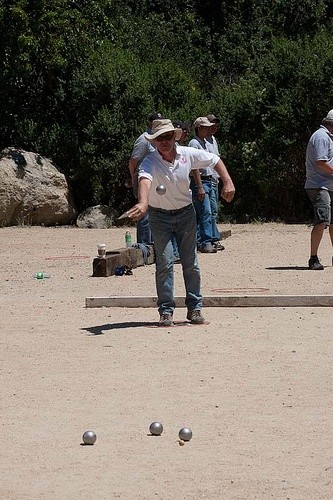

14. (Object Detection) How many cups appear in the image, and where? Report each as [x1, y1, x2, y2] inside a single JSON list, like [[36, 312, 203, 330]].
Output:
[[97, 244, 106, 258]]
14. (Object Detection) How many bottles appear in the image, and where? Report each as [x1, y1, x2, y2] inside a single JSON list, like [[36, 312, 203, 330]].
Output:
[[30, 272, 50, 278], [125, 230, 131, 248]]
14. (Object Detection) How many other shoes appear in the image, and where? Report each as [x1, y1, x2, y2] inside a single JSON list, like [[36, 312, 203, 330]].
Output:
[[308, 257, 323, 270], [173, 256, 181, 264], [211, 241, 225, 250], [201, 244, 217, 253]]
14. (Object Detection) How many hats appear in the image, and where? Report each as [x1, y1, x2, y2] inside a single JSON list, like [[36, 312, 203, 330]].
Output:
[[326, 110, 333, 119], [148, 113, 161, 121], [206, 114, 220, 123], [172, 120, 187, 130], [144, 118, 183, 143], [193, 117, 215, 128]]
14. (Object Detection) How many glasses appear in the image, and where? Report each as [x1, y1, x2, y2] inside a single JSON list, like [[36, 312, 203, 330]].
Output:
[[156, 135, 171, 142]]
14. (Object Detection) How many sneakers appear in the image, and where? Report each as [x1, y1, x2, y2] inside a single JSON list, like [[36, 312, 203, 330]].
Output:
[[159, 314, 174, 327], [186, 310, 205, 324]]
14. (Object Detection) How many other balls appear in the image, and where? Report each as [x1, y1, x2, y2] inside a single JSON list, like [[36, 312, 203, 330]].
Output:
[[155, 184, 167, 195], [82, 430, 97, 445], [179, 427, 192, 441], [150, 421, 164, 436]]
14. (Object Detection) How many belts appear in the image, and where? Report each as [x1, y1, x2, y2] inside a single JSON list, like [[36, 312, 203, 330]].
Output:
[[200, 175, 212, 180], [152, 204, 193, 216]]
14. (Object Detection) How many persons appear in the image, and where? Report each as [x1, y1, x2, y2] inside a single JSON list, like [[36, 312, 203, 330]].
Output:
[[304, 109, 333, 270], [170, 121, 188, 264], [188, 117, 217, 253], [129, 113, 162, 244], [207, 115, 225, 251], [128, 119, 235, 327]]
